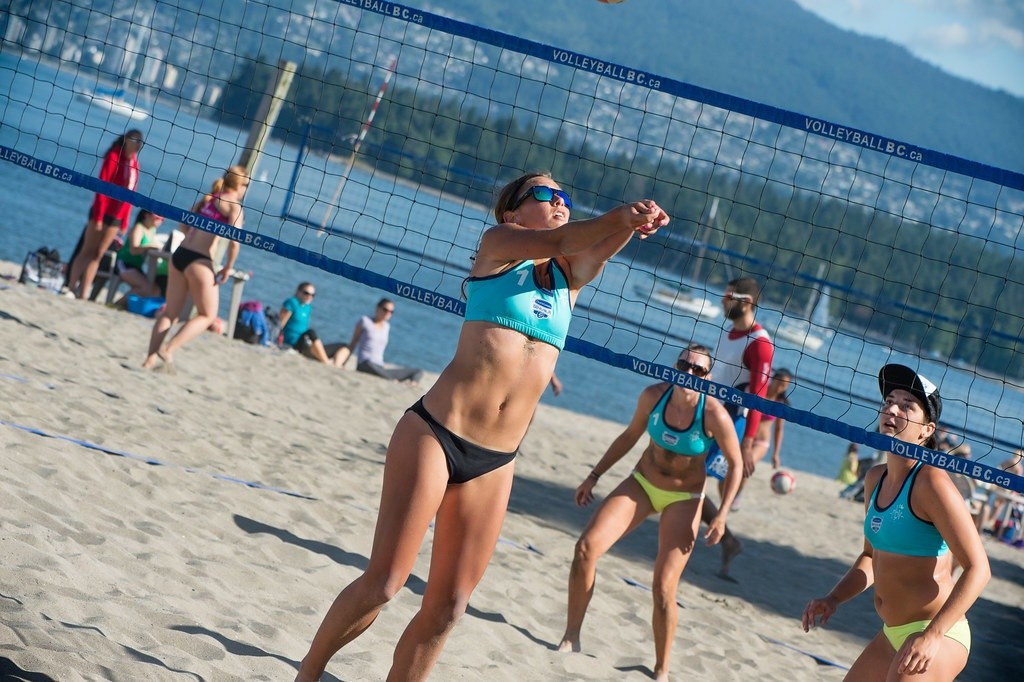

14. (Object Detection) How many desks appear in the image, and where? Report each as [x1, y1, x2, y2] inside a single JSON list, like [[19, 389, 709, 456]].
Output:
[[146, 248, 250, 340]]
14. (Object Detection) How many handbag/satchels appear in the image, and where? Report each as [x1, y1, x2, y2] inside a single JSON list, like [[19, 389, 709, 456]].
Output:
[[20, 248, 70, 293]]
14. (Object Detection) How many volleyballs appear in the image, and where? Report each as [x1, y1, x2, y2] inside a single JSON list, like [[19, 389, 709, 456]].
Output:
[[207, 318, 223, 334], [771, 470, 796, 494]]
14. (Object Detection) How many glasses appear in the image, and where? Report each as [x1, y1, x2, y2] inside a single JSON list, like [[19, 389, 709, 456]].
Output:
[[303, 291, 315, 297], [383, 307, 393, 314], [676, 359, 707, 377], [724, 293, 752, 302], [510, 186, 573, 212]]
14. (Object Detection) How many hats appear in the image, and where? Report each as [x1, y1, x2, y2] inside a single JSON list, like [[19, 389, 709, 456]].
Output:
[[878, 364, 943, 421]]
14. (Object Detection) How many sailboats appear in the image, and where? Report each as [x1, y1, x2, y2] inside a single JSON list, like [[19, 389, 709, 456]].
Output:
[[761, 262, 832, 352], [632, 196, 735, 320], [77, 14, 153, 121]]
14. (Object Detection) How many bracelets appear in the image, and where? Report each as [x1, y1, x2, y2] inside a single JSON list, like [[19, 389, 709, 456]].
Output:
[[589, 470, 600, 480]]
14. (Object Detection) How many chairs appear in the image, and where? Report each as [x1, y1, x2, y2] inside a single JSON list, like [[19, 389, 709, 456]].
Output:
[[90, 248, 123, 309]]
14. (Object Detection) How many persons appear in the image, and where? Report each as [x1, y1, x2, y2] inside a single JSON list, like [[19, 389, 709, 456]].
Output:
[[557, 342, 744, 682], [803, 364, 1024, 682], [290, 170, 669, 682], [143, 165, 251, 371], [269, 281, 424, 389], [701, 277, 791, 583], [62, 129, 161, 312], [838, 443, 861, 486]]
